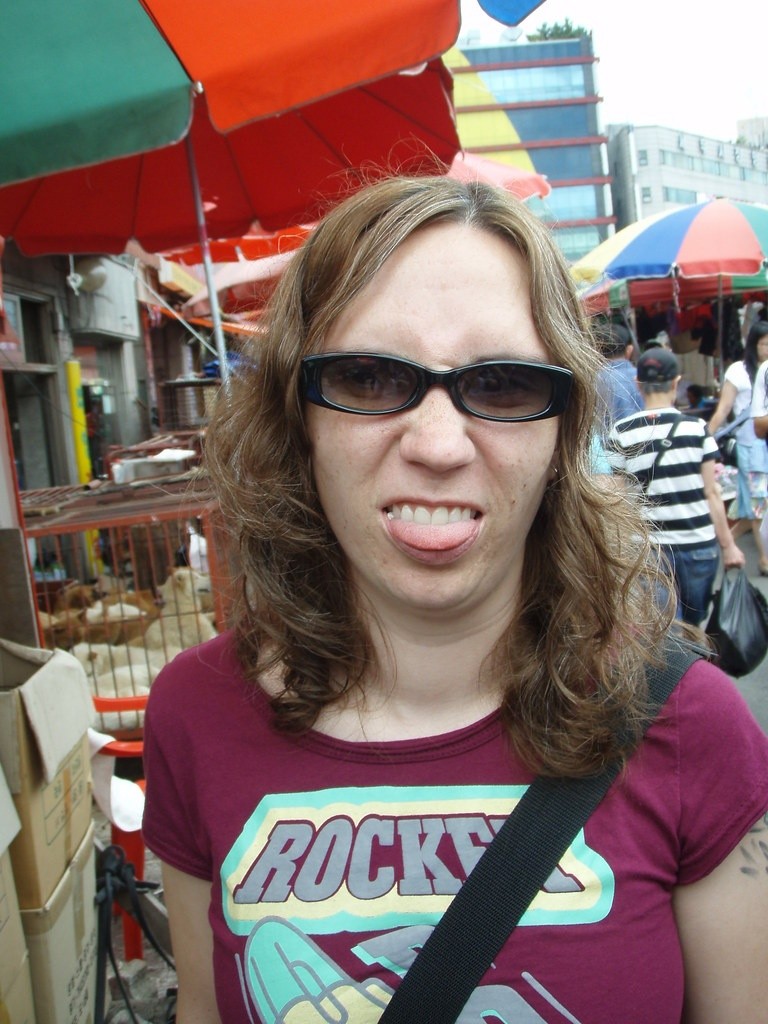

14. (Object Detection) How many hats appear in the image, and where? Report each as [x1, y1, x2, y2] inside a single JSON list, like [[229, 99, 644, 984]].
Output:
[[637, 348, 680, 382]]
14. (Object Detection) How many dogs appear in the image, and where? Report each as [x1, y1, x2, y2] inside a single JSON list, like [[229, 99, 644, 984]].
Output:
[[35, 567, 220, 739]]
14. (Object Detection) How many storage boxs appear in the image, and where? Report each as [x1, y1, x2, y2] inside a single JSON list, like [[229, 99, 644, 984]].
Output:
[[0, 638, 111, 1023]]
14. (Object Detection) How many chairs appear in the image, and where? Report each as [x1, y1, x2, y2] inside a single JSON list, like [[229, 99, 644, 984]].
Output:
[[88, 693, 151, 961]]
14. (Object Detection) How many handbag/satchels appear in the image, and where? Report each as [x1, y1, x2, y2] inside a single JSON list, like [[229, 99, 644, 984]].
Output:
[[701, 567, 768, 677]]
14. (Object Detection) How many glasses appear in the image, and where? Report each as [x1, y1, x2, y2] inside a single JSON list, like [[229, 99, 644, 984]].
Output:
[[292, 351, 575, 423]]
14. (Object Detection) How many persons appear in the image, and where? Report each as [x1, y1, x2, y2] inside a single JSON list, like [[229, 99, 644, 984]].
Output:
[[706, 320, 768, 576], [591, 323, 645, 439], [141, 175, 768, 1024], [750, 358, 768, 438], [603, 346, 746, 637]]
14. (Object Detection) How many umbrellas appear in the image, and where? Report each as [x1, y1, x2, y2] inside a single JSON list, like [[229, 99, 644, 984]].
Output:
[[570, 199, 768, 356], [0, 0, 548, 394]]
